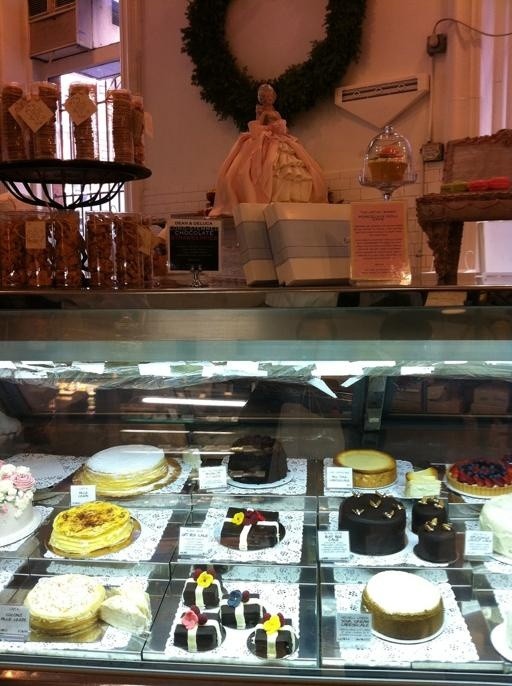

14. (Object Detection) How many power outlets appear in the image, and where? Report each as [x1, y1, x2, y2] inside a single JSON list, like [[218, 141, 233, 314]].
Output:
[[427, 33, 447, 54]]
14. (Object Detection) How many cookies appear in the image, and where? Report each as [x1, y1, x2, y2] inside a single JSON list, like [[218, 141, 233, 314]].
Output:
[[0, 85, 145, 164], [0, 214, 142, 289]]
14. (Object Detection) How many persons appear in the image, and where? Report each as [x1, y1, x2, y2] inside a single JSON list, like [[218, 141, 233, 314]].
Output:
[[208, 83, 329, 219]]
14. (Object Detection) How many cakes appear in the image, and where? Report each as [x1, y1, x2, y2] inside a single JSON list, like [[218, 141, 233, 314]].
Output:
[[173, 567, 299, 660], [227, 435, 287, 484], [0, 459, 37, 538], [214, 506, 285, 551], [22, 573, 150, 637], [48, 500, 133, 557], [367, 144, 409, 183], [334, 449, 512, 564], [359, 569, 445, 640], [81, 443, 170, 492]]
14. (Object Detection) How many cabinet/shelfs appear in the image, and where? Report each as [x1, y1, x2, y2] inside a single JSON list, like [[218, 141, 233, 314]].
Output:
[[2, 307, 511, 685]]
